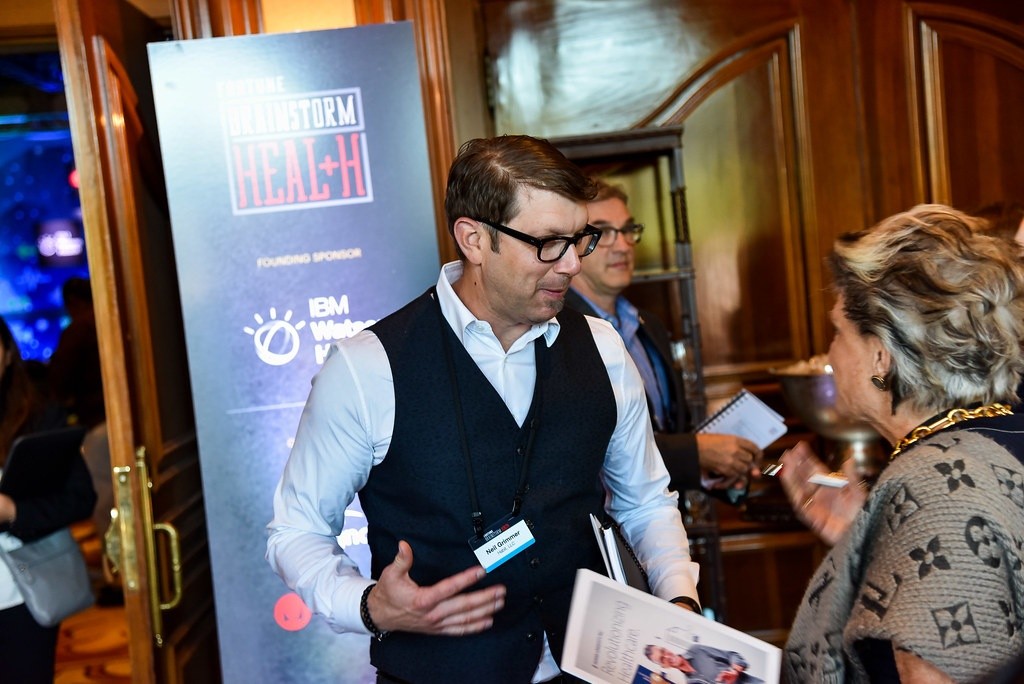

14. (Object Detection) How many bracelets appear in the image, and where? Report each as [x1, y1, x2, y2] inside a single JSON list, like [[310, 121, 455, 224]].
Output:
[[361, 584, 391, 642], [669, 596, 702, 615]]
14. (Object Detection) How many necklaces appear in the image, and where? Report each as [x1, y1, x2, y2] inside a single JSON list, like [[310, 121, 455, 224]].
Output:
[[891, 398, 1014, 459]]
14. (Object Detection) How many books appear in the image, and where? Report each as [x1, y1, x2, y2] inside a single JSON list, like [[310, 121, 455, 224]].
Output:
[[589, 514, 652, 594], [692, 387, 788, 488], [560, 568, 782, 684]]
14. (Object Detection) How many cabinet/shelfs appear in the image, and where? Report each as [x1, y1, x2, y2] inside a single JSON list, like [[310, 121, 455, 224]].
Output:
[[545, 120, 707, 431]]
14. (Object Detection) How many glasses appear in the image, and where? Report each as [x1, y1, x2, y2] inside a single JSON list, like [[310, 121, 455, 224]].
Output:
[[597, 224, 645, 247], [470, 216, 602, 263]]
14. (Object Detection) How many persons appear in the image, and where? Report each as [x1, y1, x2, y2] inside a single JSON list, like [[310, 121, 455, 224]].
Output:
[[42, 276, 126, 607], [0, 312, 99, 684], [265, 134, 702, 684], [564, 176, 763, 525], [778, 204, 1024, 684], [645, 645, 766, 684]]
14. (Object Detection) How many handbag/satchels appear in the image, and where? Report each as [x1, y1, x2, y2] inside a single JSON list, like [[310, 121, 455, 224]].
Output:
[[4, 528, 95, 627]]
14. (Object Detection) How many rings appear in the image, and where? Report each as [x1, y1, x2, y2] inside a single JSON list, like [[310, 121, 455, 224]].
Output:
[[803, 497, 814, 509]]
